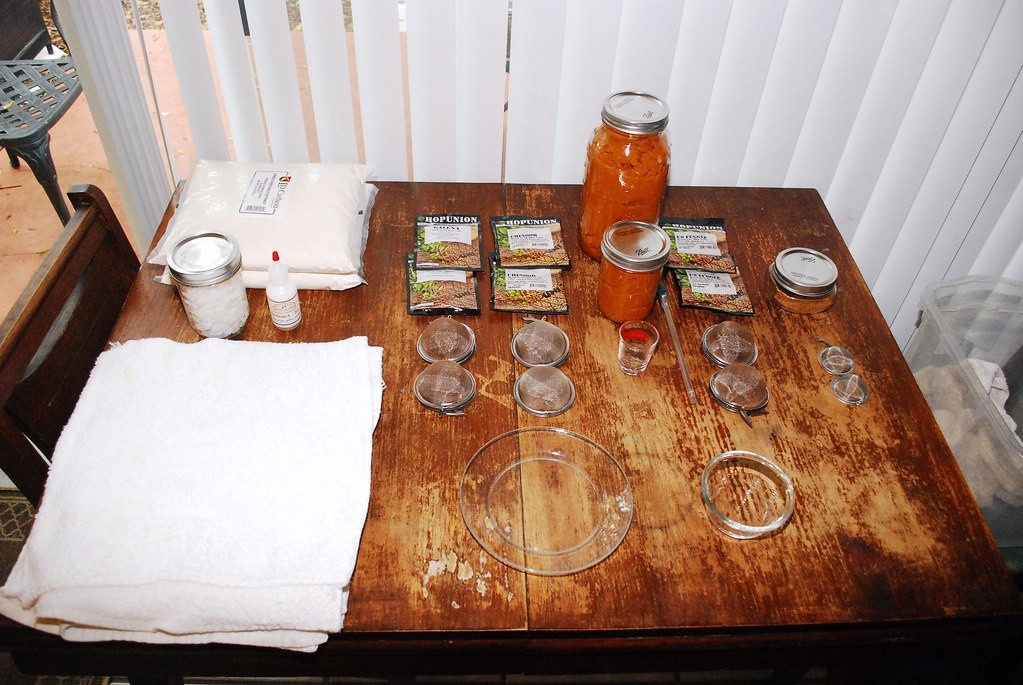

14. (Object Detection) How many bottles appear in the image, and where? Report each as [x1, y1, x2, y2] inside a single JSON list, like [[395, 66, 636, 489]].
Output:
[[264, 251, 304, 332]]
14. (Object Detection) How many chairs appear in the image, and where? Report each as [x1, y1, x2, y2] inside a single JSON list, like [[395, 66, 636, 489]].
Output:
[[0, 184, 141, 507]]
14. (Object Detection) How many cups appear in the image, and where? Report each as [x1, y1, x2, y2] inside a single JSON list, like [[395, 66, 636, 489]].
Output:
[[617, 320, 660, 376]]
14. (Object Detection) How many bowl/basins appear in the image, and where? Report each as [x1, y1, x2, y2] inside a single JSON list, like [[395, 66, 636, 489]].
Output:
[[701, 450, 796, 540]]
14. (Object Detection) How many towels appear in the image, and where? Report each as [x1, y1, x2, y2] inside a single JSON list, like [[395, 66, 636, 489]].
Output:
[[0, 336, 384, 655]]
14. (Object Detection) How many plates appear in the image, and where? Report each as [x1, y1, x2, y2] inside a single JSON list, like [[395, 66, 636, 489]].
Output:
[[459, 425, 634, 576]]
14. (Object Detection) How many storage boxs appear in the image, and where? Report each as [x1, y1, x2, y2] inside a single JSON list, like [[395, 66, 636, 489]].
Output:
[[905, 274, 1023, 580]]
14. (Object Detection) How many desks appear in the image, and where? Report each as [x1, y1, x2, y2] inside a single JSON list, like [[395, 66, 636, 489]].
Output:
[[63, 180, 1023, 685], [0, 57, 84, 227]]
